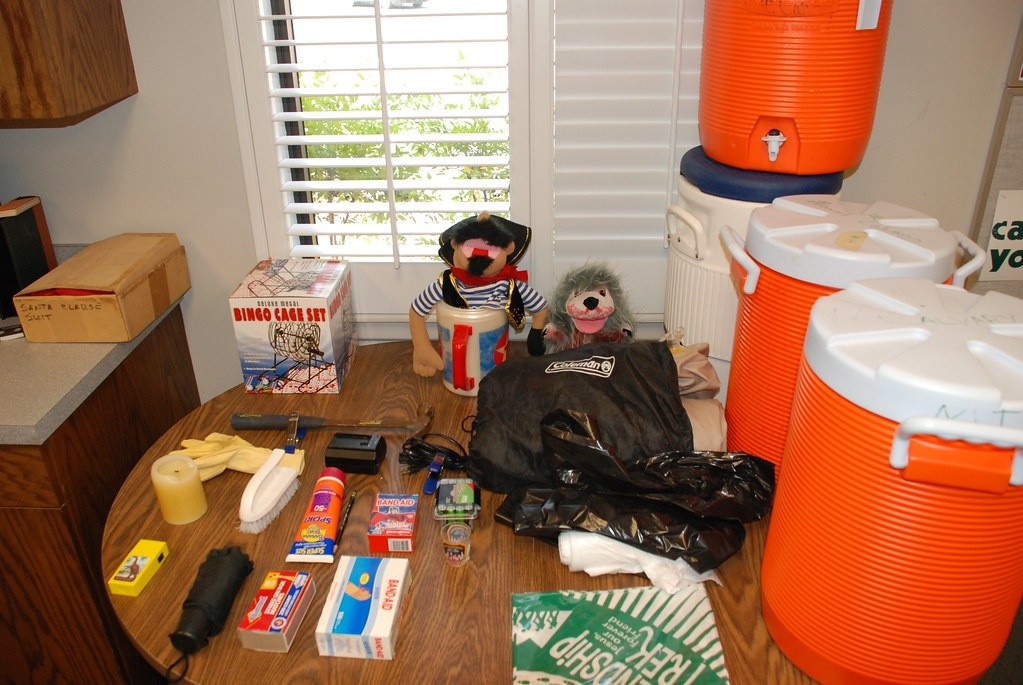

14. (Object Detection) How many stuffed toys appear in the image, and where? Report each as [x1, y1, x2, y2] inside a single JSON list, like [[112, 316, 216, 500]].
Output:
[[406, 207, 547, 377], [541, 260, 636, 359]]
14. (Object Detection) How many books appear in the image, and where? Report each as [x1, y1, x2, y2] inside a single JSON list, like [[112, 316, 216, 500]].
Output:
[[0, 198, 41, 218]]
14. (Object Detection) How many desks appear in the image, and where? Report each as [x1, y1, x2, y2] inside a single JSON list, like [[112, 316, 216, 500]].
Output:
[[101, 339, 822, 685]]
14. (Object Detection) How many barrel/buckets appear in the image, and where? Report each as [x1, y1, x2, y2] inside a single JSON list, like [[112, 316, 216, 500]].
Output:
[[759, 279, 1023, 685], [663, 144, 845, 364], [698, 0, 892, 175], [718, 194, 987, 486]]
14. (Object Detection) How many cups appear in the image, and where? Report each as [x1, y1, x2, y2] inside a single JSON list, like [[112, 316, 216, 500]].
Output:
[[440, 521, 472, 566], [434, 301, 508, 398]]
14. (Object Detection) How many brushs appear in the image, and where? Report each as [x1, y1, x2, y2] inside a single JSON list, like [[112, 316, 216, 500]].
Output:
[[232, 448, 302, 535]]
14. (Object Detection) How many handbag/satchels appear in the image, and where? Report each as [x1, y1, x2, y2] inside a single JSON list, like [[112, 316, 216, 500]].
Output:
[[461, 340, 694, 493]]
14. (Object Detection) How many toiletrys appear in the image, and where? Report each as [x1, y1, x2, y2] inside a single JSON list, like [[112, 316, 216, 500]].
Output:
[[284, 467, 347, 564]]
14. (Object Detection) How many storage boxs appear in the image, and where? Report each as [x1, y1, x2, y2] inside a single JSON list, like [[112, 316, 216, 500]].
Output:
[[760, 274, 1022, 684], [367, 492, 419, 554], [230, 260, 360, 394], [721, 191, 987, 488], [663, 144, 844, 363], [314, 555, 413, 661], [698, 0, 893, 175], [12, 232, 192, 343]]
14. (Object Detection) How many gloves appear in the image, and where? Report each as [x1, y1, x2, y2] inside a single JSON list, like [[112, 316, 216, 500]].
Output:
[[170, 432, 305, 482]]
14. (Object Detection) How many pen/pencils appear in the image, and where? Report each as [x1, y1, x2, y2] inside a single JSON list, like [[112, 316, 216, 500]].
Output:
[[333, 490, 358, 556]]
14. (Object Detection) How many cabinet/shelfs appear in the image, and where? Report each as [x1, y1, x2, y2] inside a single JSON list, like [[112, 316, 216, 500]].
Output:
[[0, 0, 138, 131], [1, 444, 130, 684]]
